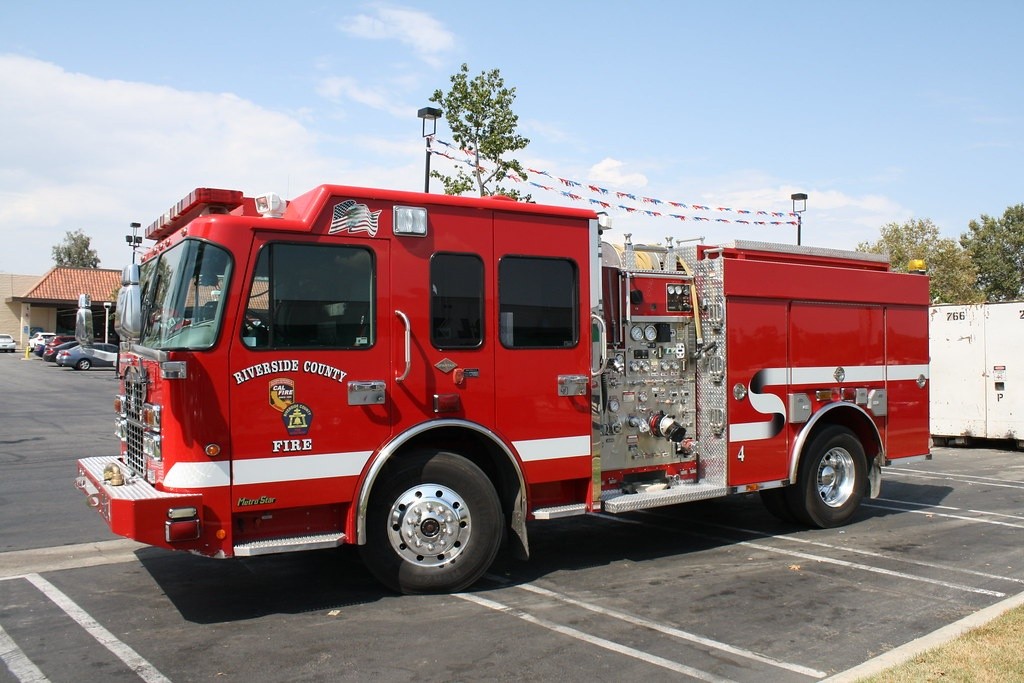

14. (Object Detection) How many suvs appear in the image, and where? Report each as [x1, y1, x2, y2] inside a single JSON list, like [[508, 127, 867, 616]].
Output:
[[44, 335, 76, 351], [29, 332, 56, 353]]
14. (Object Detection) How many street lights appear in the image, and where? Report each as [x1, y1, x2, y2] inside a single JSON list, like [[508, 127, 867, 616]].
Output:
[[790, 191, 808, 246], [417, 105, 444, 194], [103, 302, 112, 343], [126, 222, 142, 265]]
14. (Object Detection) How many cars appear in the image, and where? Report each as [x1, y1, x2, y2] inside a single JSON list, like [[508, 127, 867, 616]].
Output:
[[56, 342, 118, 371], [0, 334, 16, 353], [43, 341, 79, 367], [34, 344, 45, 357]]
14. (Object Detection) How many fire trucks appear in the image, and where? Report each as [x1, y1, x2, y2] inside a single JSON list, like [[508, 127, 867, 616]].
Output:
[[74, 185, 933, 598]]
